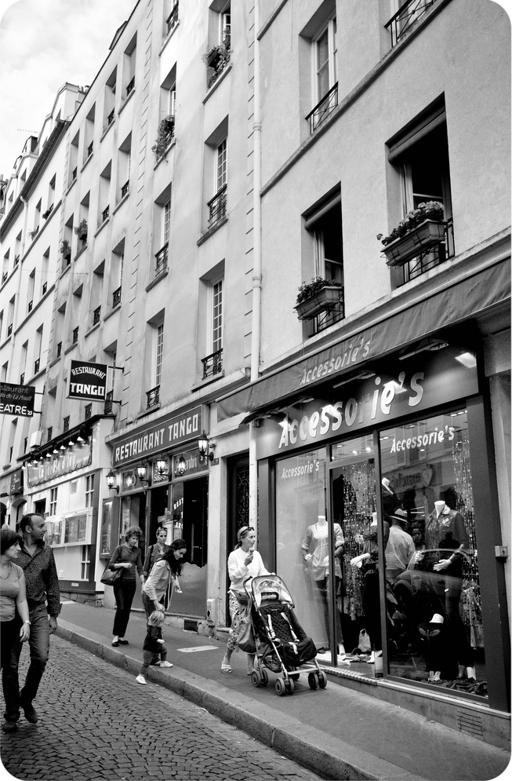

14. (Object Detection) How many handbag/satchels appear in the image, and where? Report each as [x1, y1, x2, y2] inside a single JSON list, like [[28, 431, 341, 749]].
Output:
[[236, 623, 255, 653], [100, 569, 120, 585], [238, 592, 248, 605]]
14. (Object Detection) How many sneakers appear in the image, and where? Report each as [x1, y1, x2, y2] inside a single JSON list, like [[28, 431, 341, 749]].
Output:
[[112, 639, 119, 646], [160, 660, 174, 668], [136, 674, 147, 685]]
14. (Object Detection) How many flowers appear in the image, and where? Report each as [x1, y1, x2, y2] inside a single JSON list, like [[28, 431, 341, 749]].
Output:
[[376, 200, 446, 245], [296, 275, 342, 304]]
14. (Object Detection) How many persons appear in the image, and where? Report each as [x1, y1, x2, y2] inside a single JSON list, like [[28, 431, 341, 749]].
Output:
[[222, 526, 276, 673], [301, 474, 476, 683], [108, 526, 186, 685], [0, 502, 63, 731]]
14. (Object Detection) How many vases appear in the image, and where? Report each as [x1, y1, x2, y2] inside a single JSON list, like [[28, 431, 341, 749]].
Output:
[[293, 286, 343, 320], [381, 218, 447, 266]]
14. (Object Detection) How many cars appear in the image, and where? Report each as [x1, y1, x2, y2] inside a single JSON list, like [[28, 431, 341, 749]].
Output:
[[394, 548, 480, 601]]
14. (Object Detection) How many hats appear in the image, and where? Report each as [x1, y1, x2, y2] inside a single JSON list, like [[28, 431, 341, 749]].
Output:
[[381, 477, 394, 495], [389, 508, 408, 523]]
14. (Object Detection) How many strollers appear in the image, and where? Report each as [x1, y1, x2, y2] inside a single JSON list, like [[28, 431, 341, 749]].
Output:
[[241, 571, 329, 697]]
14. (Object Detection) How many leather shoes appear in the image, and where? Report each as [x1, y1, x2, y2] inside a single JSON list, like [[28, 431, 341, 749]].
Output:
[[3, 721, 16, 731], [119, 640, 128, 644], [24, 704, 38, 723]]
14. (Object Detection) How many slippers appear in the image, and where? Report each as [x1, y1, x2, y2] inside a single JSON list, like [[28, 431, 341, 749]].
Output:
[[221, 664, 232, 673]]
